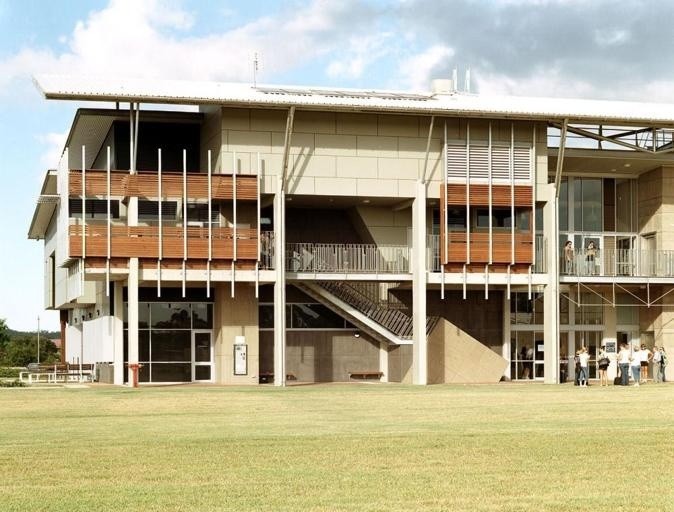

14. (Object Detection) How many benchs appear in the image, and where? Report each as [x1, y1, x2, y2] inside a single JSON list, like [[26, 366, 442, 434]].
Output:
[[260, 373, 296, 384], [348, 370, 385, 381], [19, 361, 95, 382]]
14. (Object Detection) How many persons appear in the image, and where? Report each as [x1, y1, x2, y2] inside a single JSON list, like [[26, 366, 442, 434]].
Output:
[[572, 343, 669, 387], [585, 241, 596, 275], [564, 241, 573, 276], [521, 343, 529, 375], [522, 348, 533, 379]]
